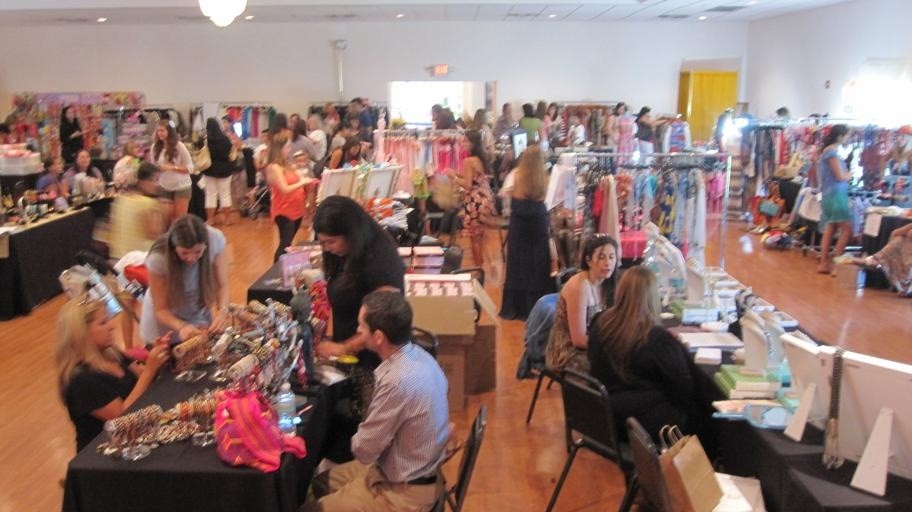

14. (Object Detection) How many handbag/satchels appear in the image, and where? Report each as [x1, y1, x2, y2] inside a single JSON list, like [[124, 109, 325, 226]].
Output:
[[656, 424, 725, 512]]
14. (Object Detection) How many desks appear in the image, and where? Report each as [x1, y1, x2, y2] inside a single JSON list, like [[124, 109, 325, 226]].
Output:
[[63, 360, 328, 512], [70, 181, 110, 217], [1, 207, 95, 315], [1, 231, 15, 321], [660, 265, 911, 511], [248, 258, 293, 306]]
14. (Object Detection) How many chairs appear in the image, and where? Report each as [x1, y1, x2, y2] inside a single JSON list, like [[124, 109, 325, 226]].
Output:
[[412, 325, 438, 360], [516, 293, 560, 423], [545, 368, 633, 512], [450, 268, 484, 323], [444, 404, 486, 512]]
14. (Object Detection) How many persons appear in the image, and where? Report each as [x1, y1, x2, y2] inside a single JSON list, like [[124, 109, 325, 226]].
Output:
[[430, 100, 912, 512], [2, 100, 457, 511]]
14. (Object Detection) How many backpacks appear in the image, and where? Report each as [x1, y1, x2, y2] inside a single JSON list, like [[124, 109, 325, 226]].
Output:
[[213, 390, 307, 475]]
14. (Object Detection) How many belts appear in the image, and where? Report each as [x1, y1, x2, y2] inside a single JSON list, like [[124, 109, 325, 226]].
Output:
[[407, 475, 436, 486]]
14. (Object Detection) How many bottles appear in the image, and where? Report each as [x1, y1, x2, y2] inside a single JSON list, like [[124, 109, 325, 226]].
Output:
[[277, 383, 296, 436]]
